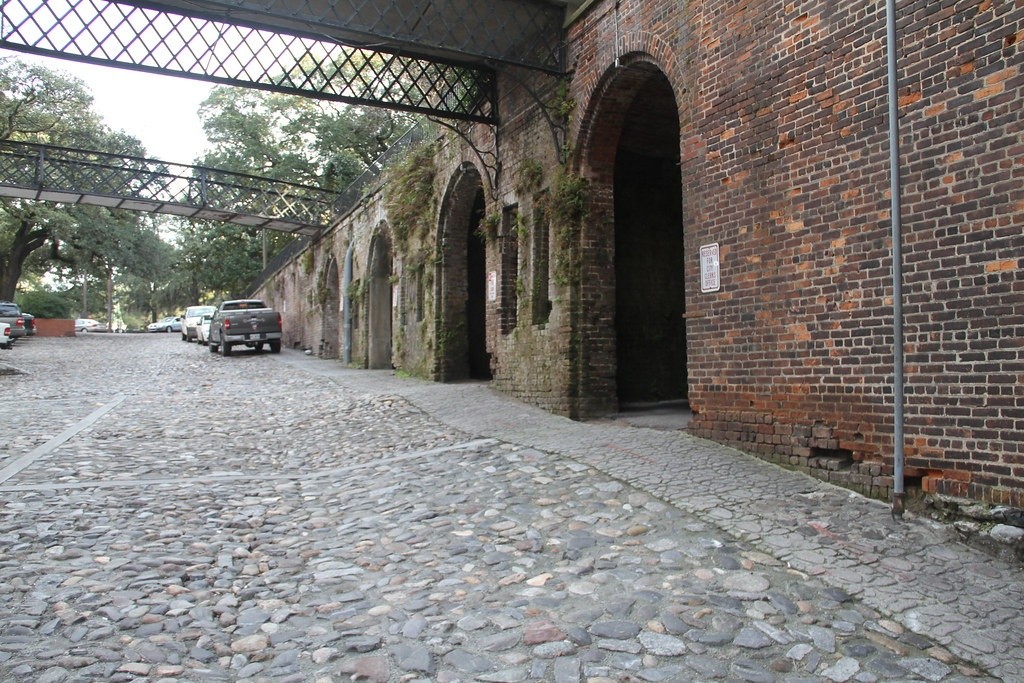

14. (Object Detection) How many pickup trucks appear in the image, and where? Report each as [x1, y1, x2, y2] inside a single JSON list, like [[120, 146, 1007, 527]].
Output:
[[203, 298, 283, 358]]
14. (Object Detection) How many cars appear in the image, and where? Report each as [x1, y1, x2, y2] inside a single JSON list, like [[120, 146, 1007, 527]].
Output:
[[74, 317, 107, 333], [21, 312, 37, 336], [196, 313, 214, 346], [0, 322, 15, 348], [147, 316, 183, 333]]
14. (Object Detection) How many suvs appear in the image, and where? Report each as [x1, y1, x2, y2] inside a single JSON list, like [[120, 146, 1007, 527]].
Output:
[[180, 306, 216, 342], [0, 299, 26, 337]]
